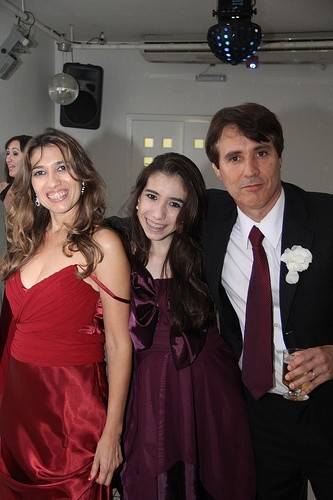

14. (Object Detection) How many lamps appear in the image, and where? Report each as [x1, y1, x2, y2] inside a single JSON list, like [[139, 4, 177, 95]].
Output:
[[195, 74, 226, 82], [207, 0, 262, 66], [47, 40, 79, 105]]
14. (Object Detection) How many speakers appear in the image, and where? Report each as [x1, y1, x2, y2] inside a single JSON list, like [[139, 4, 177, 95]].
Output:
[[60, 62, 104, 131]]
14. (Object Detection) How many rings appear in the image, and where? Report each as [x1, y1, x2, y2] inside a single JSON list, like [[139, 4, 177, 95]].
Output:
[[311, 371, 316, 378]]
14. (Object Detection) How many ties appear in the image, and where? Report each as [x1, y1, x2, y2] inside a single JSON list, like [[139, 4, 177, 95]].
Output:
[[242, 227, 274, 397]]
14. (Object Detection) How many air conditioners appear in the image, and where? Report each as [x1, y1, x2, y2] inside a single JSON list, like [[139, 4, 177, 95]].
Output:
[[141, 31, 333, 64]]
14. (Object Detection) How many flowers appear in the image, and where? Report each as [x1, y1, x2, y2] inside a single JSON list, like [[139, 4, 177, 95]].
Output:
[[280, 245, 313, 285]]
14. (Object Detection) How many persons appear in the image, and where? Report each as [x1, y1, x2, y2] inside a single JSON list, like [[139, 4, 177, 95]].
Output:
[[122, 154, 259, 500], [0, 131, 132, 500], [100, 103, 333, 500]]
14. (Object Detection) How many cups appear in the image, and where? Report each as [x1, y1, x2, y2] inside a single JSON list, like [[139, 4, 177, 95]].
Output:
[[282, 348, 312, 401]]
